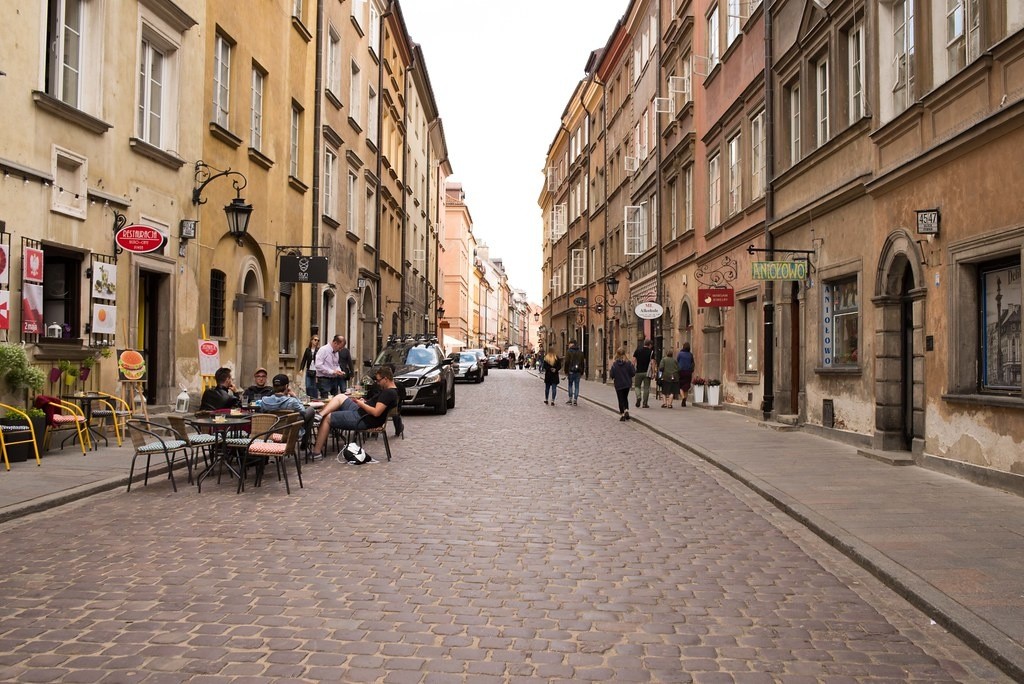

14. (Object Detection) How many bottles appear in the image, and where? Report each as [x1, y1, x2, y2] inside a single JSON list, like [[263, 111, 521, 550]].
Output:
[[175, 389, 190, 413], [48, 322, 62, 338]]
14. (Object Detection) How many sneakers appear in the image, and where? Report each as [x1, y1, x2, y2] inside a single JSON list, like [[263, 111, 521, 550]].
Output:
[[305, 451, 323, 461], [312, 411, 322, 428]]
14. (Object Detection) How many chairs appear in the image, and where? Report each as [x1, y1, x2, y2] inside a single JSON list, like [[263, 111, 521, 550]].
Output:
[[0, 392, 390, 495]]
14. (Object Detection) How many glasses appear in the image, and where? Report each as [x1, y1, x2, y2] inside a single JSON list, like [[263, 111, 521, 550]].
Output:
[[311, 339, 319, 342], [374, 376, 386, 382], [255, 376, 267, 379]]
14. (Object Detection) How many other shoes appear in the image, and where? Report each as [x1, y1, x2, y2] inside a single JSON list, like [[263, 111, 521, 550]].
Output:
[[681, 398, 686, 407], [544, 401, 548, 404], [566, 401, 572, 405], [625, 412, 630, 420], [643, 402, 649, 408], [668, 405, 672, 408], [550, 402, 554, 406], [574, 402, 576, 405], [620, 416, 625, 421], [661, 404, 667, 408], [636, 399, 640, 407]]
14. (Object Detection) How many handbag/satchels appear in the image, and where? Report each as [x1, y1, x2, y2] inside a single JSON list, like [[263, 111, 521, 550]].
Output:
[[645, 359, 657, 380]]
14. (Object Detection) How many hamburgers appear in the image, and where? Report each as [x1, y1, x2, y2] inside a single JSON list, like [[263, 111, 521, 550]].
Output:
[[118, 350, 145, 379]]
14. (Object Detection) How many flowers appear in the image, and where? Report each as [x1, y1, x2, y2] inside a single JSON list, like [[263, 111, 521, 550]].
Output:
[[691, 375, 705, 384], [0, 343, 28, 374], [6, 365, 47, 394]]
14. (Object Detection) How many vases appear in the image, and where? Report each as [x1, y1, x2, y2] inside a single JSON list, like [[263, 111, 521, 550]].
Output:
[[15, 380, 30, 389], [692, 385, 704, 403]]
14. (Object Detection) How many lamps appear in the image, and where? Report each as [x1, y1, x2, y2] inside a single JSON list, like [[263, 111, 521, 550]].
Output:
[[428, 298, 445, 320], [192, 160, 254, 243], [606, 265, 631, 294]]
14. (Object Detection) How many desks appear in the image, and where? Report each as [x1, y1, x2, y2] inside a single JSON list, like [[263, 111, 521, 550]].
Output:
[[190, 418, 251, 494], [201, 410, 258, 477], [61, 393, 110, 451]]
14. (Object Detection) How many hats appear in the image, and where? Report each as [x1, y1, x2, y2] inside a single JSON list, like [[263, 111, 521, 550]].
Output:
[[566, 340, 576, 346], [616, 347, 625, 355], [254, 367, 267, 376], [273, 374, 289, 386]]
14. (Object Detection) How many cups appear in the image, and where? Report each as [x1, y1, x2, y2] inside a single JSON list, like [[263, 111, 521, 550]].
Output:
[[306, 396, 310, 402], [231, 408, 241, 414], [254, 394, 261, 400], [351, 389, 355, 396], [214, 413, 225, 421], [328, 395, 333, 400], [242, 396, 248, 407]]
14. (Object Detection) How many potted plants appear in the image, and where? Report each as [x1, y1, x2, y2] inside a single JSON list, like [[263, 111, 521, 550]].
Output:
[[707, 379, 721, 406], [50, 361, 71, 383], [80, 356, 95, 380], [28, 408, 47, 459], [0, 406, 29, 463], [65, 369, 78, 386]]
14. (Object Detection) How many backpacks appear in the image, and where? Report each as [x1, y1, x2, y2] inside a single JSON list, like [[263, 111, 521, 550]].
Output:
[[337, 442, 371, 465], [568, 349, 581, 373]]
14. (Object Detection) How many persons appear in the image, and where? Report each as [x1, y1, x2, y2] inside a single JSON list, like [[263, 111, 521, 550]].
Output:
[[632, 339, 655, 408], [299, 334, 321, 399], [543, 346, 563, 406], [315, 335, 346, 399], [331, 348, 354, 394], [658, 349, 679, 408], [261, 374, 305, 437], [564, 340, 584, 406], [676, 342, 695, 407], [610, 347, 635, 421], [508, 349, 545, 373], [199, 368, 240, 409], [242, 368, 274, 403], [309, 362, 398, 460]]
